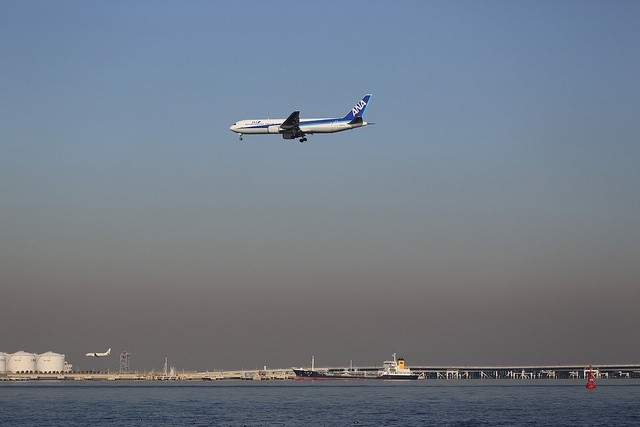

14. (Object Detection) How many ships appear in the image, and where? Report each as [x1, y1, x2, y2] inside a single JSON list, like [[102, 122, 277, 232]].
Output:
[[292, 351, 418, 380]]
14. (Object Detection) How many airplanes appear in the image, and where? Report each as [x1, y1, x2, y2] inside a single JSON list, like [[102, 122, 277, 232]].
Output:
[[229, 92, 377, 144], [86, 347, 111, 356]]
